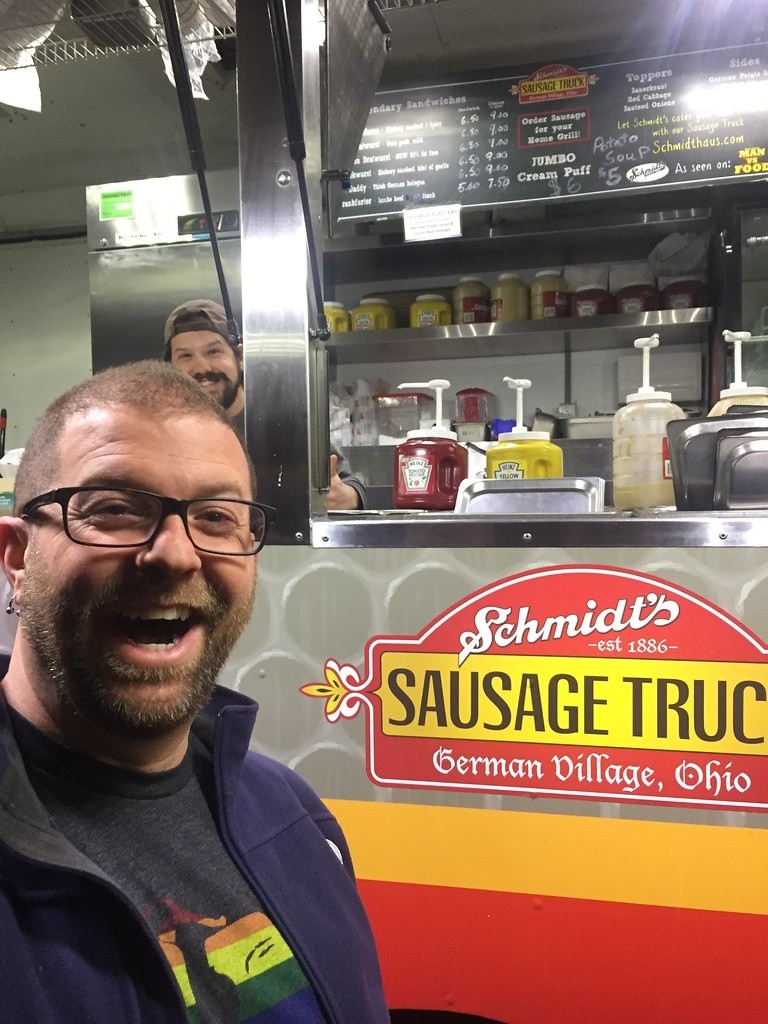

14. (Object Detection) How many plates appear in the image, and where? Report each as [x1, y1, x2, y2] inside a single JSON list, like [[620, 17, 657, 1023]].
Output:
[[666, 414, 768, 511], [454, 477, 606, 514]]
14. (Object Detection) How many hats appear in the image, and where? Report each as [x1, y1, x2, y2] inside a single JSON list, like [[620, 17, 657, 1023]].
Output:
[[164, 298, 238, 361]]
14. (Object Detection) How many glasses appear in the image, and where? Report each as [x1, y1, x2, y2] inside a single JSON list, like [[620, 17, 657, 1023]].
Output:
[[19, 486, 276, 556]]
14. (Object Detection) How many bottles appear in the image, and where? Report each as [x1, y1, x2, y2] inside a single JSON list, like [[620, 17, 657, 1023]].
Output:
[[707, 329, 768, 417], [323, 271, 710, 333], [612, 333, 686, 511], [394, 379, 469, 510], [486, 376, 563, 479]]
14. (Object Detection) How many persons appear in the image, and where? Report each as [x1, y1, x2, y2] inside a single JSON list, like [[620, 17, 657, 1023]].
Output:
[[162, 300, 369, 514], [0, 360, 392, 1024]]
